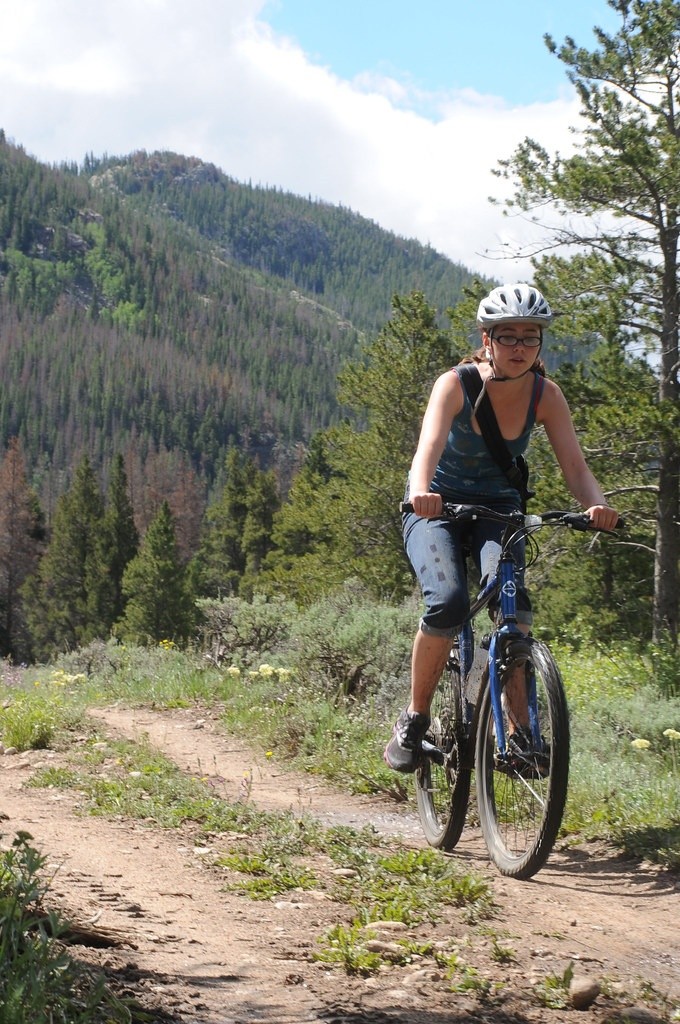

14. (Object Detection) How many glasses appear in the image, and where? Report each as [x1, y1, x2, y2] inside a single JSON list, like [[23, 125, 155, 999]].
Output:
[[484, 330, 544, 348]]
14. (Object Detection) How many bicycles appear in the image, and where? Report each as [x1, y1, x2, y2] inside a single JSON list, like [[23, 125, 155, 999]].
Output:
[[397, 492, 626, 881]]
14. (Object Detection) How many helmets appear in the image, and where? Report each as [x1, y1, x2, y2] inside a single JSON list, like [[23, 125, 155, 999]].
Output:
[[476, 284, 553, 332]]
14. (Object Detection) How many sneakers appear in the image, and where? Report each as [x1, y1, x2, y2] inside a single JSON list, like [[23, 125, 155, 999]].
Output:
[[383, 704, 429, 769], [500, 724, 550, 777]]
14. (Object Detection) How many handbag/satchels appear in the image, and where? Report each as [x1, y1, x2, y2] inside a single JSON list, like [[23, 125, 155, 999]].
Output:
[[511, 450, 534, 516]]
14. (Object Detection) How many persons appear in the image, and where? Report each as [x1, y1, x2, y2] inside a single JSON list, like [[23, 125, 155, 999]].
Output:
[[383, 284, 619, 782]]
